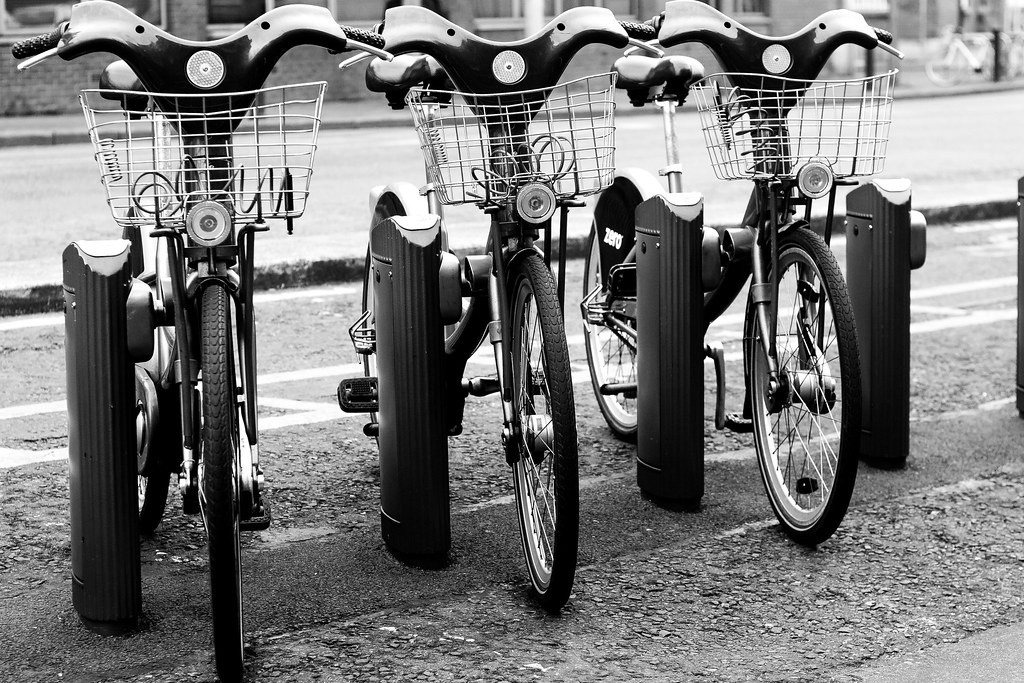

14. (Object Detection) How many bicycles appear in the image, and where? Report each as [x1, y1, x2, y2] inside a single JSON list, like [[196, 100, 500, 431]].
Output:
[[10, 0, 394, 682], [328, 4, 665, 615], [575, 0, 905, 547]]
